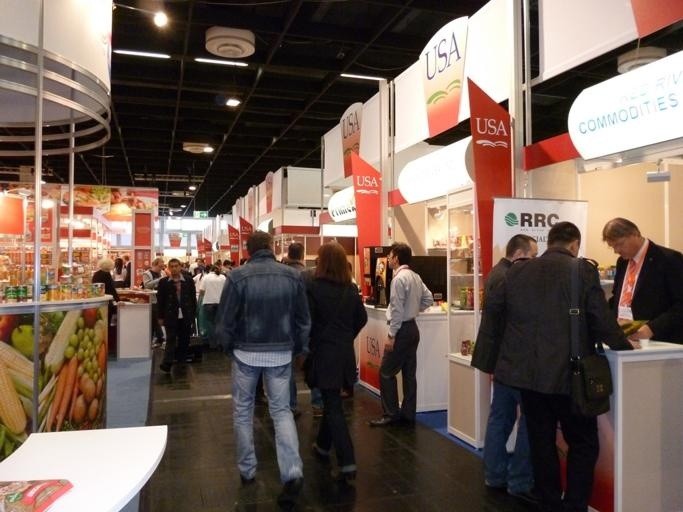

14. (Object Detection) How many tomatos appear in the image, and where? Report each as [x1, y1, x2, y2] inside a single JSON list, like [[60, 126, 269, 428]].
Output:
[[0, 314, 20, 339], [84, 308, 97, 323]]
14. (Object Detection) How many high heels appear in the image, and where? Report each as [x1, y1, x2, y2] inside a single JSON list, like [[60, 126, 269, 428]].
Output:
[[311, 443, 329, 458], [330, 469, 356, 480]]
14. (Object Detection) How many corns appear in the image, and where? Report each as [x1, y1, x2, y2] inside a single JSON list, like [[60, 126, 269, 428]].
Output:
[[0, 360, 27, 435], [0, 340, 42, 391], [44, 309, 82, 375]]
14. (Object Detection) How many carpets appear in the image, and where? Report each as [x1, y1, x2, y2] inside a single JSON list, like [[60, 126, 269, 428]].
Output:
[[102, 356, 153, 512]]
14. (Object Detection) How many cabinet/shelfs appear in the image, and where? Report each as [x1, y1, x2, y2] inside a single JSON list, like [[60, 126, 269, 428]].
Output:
[[57, 204, 112, 283], [445, 184, 487, 449]]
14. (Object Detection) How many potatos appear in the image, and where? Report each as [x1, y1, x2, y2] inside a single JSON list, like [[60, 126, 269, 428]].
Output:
[[73, 374, 103, 424]]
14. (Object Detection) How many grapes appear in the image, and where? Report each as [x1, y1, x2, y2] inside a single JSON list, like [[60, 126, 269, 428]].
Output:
[[64, 316, 103, 383]]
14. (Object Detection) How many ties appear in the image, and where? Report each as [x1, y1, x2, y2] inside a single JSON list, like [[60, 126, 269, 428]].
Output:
[[620, 259, 637, 306]]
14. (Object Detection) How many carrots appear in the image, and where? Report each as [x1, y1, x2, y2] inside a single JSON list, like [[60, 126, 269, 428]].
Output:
[[47, 355, 84, 432]]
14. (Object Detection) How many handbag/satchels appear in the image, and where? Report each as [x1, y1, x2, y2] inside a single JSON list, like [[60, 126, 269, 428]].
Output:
[[570, 353, 612, 416]]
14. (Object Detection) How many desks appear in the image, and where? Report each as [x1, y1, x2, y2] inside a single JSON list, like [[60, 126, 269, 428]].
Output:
[[0, 421, 169, 512], [112, 302, 154, 363]]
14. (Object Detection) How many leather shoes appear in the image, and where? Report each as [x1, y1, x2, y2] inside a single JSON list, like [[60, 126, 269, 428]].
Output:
[[369, 415, 415, 427]]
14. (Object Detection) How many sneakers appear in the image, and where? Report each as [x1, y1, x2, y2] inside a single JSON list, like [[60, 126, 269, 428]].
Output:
[[278, 478, 302, 508], [506, 488, 540, 505], [257, 396, 268, 407], [313, 406, 326, 418], [160, 363, 170, 372]]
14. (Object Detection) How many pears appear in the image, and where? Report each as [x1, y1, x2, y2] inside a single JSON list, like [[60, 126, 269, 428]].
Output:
[[11, 325, 45, 360]]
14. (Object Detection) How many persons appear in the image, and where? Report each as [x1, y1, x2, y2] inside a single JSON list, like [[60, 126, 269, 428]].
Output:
[[302, 242, 369, 475], [92, 253, 131, 328], [467, 233, 539, 503], [212, 229, 312, 504], [493, 220, 641, 512], [601, 217, 683, 345], [141, 243, 361, 417], [369, 242, 434, 429]]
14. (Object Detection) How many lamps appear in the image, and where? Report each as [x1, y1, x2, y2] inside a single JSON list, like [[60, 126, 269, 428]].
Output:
[[179, 141, 212, 155], [203, 26, 257, 59], [613, 45, 667, 74]]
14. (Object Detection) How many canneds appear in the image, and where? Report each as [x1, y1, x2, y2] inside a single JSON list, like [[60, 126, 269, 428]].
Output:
[[6, 267, 106, 302]]
